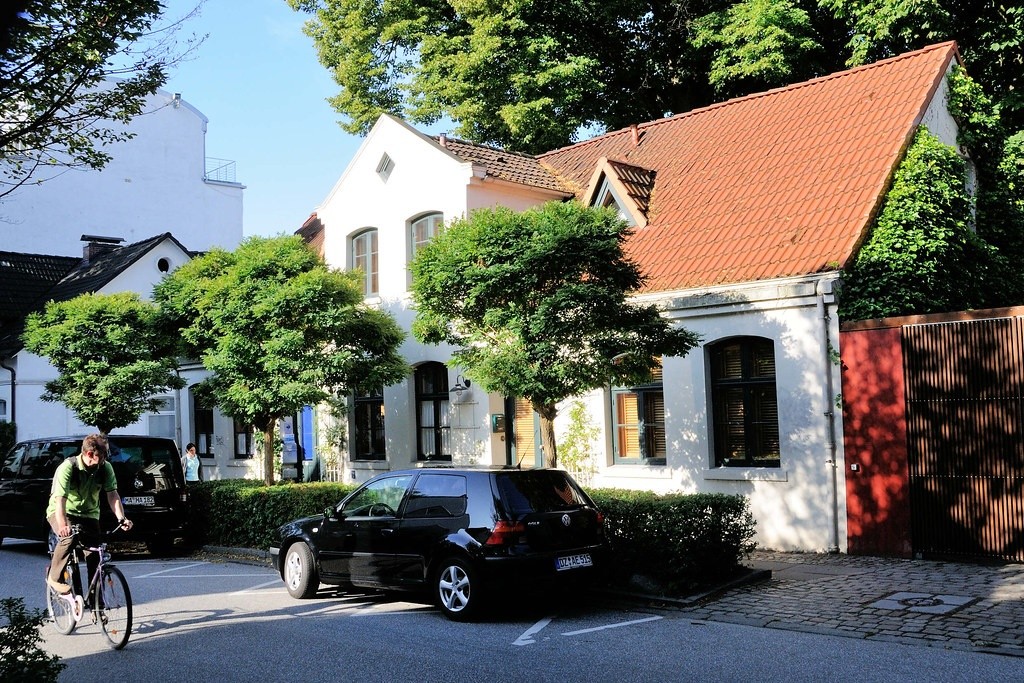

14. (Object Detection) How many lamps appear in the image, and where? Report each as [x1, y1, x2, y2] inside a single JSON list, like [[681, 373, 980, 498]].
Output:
[[449, 374, 474, 396]]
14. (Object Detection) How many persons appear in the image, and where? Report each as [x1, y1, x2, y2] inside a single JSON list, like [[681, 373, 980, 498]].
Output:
[[179, 443, 205, 486], [46, 433, 135, 624]]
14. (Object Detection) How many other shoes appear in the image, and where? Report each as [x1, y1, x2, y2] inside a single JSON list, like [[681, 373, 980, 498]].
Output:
[[48, 574, 71, 594], [90, 612, 108, 625]]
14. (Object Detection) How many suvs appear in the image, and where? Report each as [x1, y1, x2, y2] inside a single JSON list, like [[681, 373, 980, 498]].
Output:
[[0, 434, 193, 559]]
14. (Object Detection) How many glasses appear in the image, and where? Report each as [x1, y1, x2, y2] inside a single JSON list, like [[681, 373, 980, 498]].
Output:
[[101, 449, 112, 458]]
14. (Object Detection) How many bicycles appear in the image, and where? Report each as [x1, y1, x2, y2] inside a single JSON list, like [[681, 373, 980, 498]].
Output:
[[46, 517, 133, 650]]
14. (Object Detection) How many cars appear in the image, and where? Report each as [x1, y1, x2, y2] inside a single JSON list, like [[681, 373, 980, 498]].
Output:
[[269, 462, 609, 622]]
